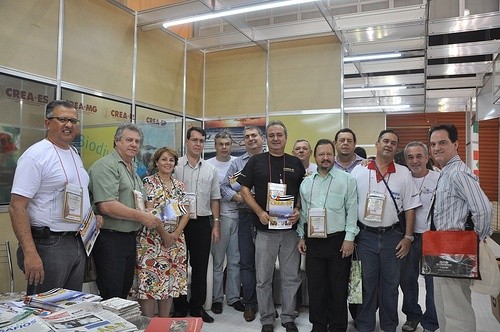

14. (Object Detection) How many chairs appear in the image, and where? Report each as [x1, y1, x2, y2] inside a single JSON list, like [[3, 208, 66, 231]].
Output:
[[0, 240, 14, 293]]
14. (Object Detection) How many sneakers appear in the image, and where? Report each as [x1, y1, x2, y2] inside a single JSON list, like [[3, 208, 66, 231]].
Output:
[[402, 318, 420, 332], [423, 329, 435, 332]]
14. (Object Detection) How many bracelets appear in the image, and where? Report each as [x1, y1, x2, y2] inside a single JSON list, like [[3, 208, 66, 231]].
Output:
[[214, 218, 219, 221], [405, 235, 414, 241], [298, 236, 304, 239]]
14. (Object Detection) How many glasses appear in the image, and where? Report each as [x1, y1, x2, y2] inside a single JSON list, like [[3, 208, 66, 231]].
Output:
[[49, 117, 77, 124], [189, 138, 204, 143]]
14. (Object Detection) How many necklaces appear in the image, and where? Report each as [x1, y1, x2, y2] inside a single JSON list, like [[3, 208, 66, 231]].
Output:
[[418, 176, 425, 191]]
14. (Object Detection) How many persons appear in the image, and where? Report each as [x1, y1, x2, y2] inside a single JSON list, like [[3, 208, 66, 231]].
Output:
[[332, 128, 363, 175], [291, 140, 317, 176], [220, 126, 279, 321], [237, 122, 306, 332], [87, 123, 163, 302], [350, 130, 423, 332], [429, 125, 493, 332], [296, 139, 360, 332], [9, 99, 105, 295], [128, 147, 189, 318], [173, 127, 221, 323], [360, 142, 440, 332], [207, 131, 244, 314]]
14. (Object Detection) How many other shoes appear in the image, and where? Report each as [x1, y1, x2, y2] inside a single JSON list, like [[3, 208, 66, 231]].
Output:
[[282, 322, 298, 332], [262, 324, 273, 332], [295, 310, 300, 316]]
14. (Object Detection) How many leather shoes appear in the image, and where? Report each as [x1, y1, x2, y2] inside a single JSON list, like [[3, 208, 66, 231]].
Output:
[[244, 307, 255, 321], [229, 301, 245, 311], [190, 303, 214, 322], [211, 302, 222, 314], [174, 312, 187, 317], [276, 310, 278, 318]]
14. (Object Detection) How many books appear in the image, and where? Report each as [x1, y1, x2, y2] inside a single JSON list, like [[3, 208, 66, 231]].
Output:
[[74, 205, 100, 257], [24, 288, 145, 330]]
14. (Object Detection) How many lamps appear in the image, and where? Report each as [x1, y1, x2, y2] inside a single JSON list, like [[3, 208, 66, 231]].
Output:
[[344, 85, 409, 92], [344, 52, 405, 63], [141, 0, 319, 32], [344, 105, 412, 111]]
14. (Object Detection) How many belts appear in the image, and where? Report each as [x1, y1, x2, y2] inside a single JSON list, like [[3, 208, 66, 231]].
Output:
[[327, 231, 345, 238], [239, 208, 253, 215], [412, 233, 422, 238], [98, 228, 138, 235], [49, 230, 80, 238], [357, 220, 400, 233]]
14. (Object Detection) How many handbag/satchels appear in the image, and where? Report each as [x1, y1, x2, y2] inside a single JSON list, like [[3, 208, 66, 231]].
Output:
[[420, 231, 481, 280], [471, 239, 500, 300], [346, 246, 363, 305], [84, 253, 97, 282], [398, 212, 406, 235]]
